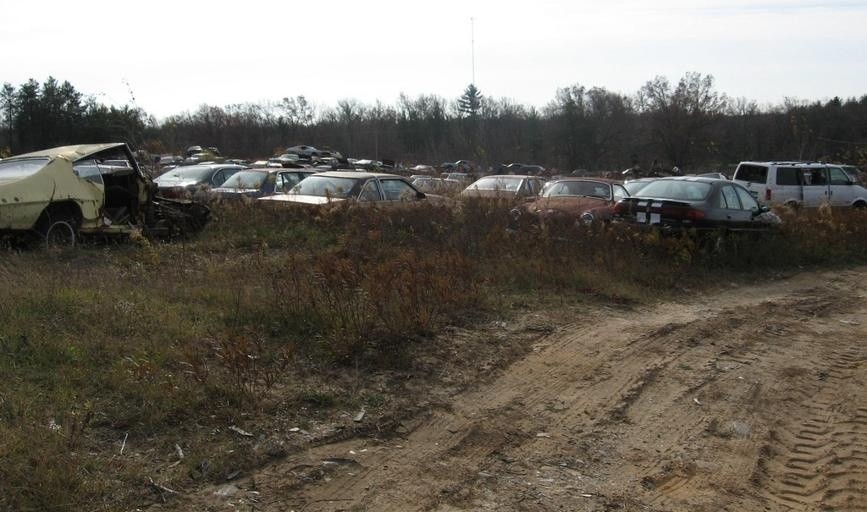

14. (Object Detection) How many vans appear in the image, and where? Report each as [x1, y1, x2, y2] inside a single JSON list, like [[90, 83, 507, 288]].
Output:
[[732, 161, 867, 220]]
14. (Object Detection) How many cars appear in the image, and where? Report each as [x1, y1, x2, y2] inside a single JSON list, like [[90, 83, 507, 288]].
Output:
[[78, 146, 729, 214], [254, 170, 454, 231], [830, 163, 864, 186], [612, 173, 783, 241]]
